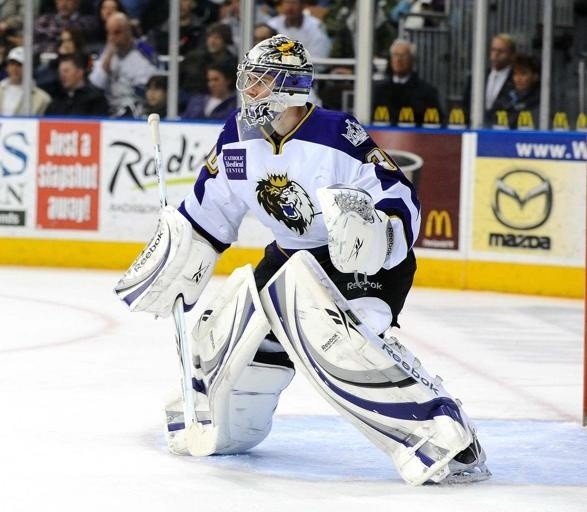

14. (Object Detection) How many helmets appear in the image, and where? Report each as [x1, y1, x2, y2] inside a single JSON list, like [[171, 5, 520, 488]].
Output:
[[235, 34, 314, 113]]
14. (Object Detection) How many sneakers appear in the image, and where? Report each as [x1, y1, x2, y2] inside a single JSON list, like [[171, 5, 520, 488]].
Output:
[[450, 438, 487, 472]]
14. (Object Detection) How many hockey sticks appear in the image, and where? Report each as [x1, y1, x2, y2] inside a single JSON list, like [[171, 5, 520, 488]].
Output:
[[148, 113, 233, 457]]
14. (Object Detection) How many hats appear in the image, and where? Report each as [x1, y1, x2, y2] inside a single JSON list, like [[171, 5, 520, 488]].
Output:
[[8, 46, 25, 64]]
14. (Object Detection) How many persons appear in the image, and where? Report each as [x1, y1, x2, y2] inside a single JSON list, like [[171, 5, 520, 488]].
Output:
[[111, 32, 493, 482], [1, 1, 443, 121], [491, 54, 539, 126], [463, 31, 518, 124]]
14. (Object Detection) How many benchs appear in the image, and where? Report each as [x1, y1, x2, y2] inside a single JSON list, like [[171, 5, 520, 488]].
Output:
[[39, 50, 391, 84]]
